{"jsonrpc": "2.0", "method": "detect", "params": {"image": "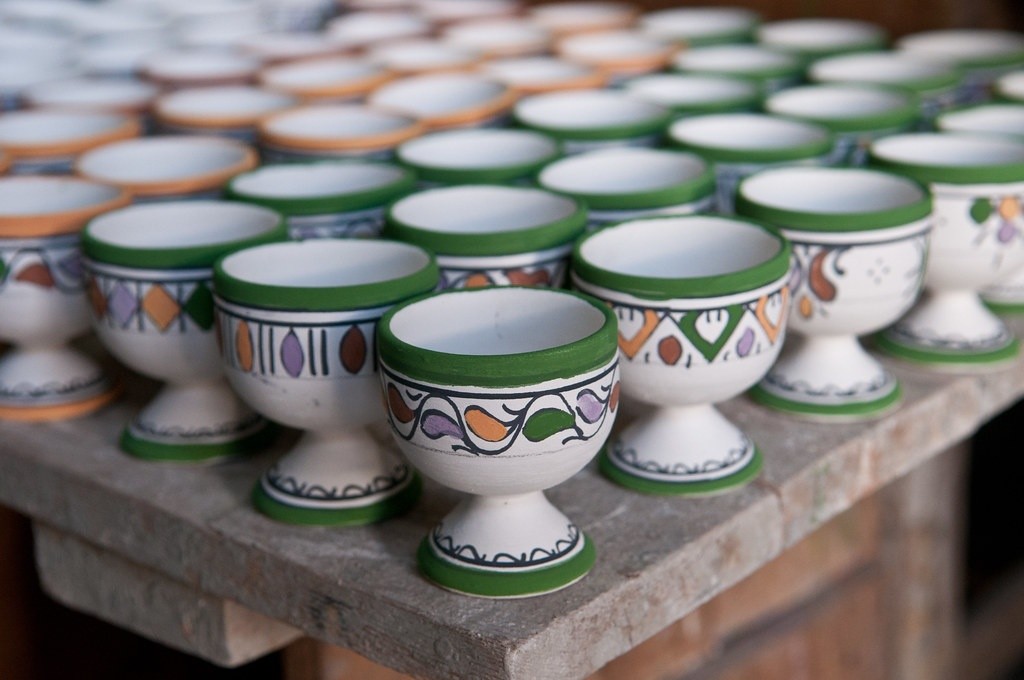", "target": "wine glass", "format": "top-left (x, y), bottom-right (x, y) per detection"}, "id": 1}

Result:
top-left (0, 0), bottom-right (1024, 599)
